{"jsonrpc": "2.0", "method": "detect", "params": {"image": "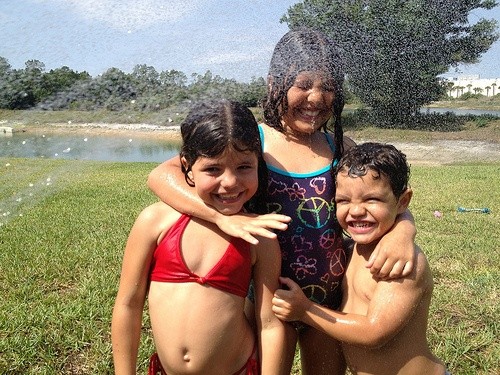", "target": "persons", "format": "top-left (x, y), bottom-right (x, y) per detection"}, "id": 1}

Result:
top-left (146, 27), bottom-right (417, 375)
top-left (271, 142), bottom-right (446, 375)
top-left (111, 99), bottom-right (285, 375)
